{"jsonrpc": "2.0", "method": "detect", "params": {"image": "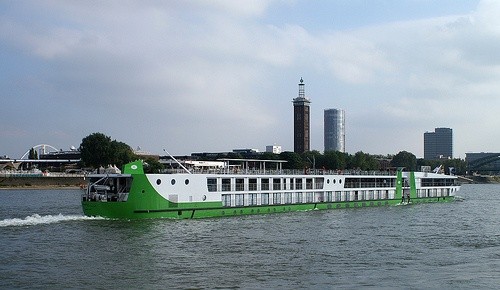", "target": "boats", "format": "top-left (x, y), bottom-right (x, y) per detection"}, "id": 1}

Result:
top-left (80, 162), bottom-right (461, 220)
